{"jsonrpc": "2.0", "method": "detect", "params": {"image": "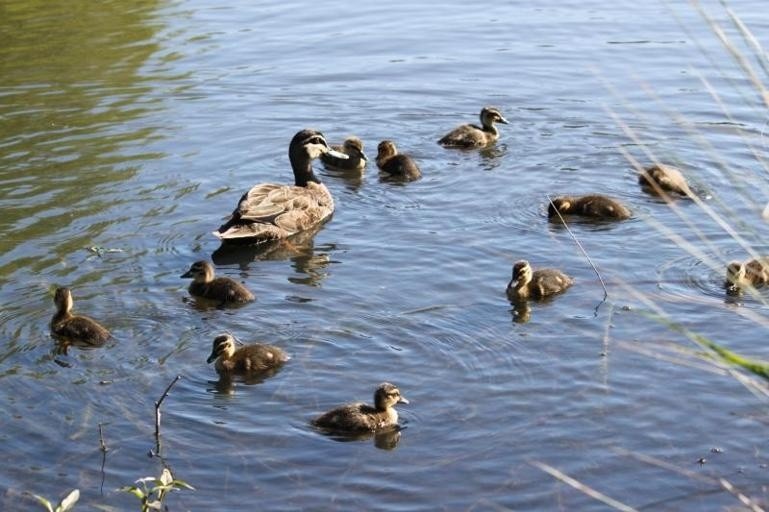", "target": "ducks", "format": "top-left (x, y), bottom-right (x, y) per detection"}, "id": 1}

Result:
top-left (638, 164), bottom-right (704, 200)
top-left (206, 372), bottom-right (279, 396)
top-left (546, 194), bottom-right (632, 221)
top-left (725, 256), bottom-right (768, 292)
top-left (505, 298), bottom-right (559, 324)
top-left (319, 135), bottom-right (422, 180)
top-left (311, 382), bottom-right (410, 433)
top-left (329, 423), bottom-right (406, 450)
top-left (49, 285), bottom-right (114, 350)
top-left (180, 259), bottom-right (257, 305)
top-left (204, 332), bottom-right (293, 373)
top-left (505, 260), bottom-right (574, 298)
top-left (435, 105), bottom-right (511, 150)
top-left (210, 127), bottom-right (351, 247)
